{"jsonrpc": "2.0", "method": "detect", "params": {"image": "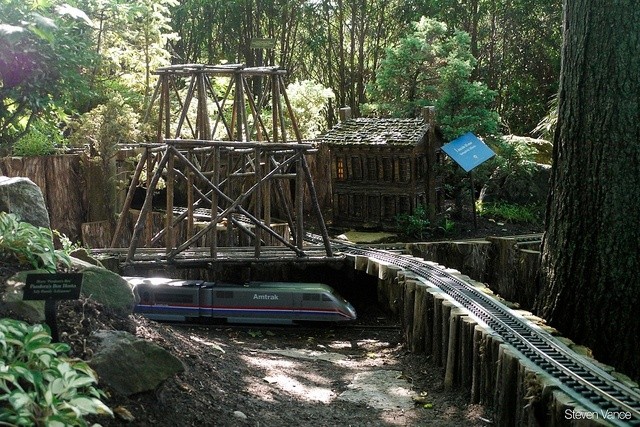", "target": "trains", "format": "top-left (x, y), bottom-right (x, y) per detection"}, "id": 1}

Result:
top-left (118, 276), bottom-right (357, 325)
